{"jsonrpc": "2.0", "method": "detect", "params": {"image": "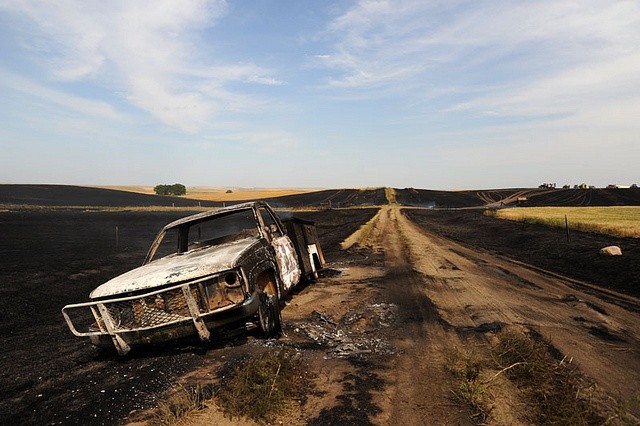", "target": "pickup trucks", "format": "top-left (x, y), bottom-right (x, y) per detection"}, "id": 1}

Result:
top-left (63, 202), bottom-right (328, 355)
